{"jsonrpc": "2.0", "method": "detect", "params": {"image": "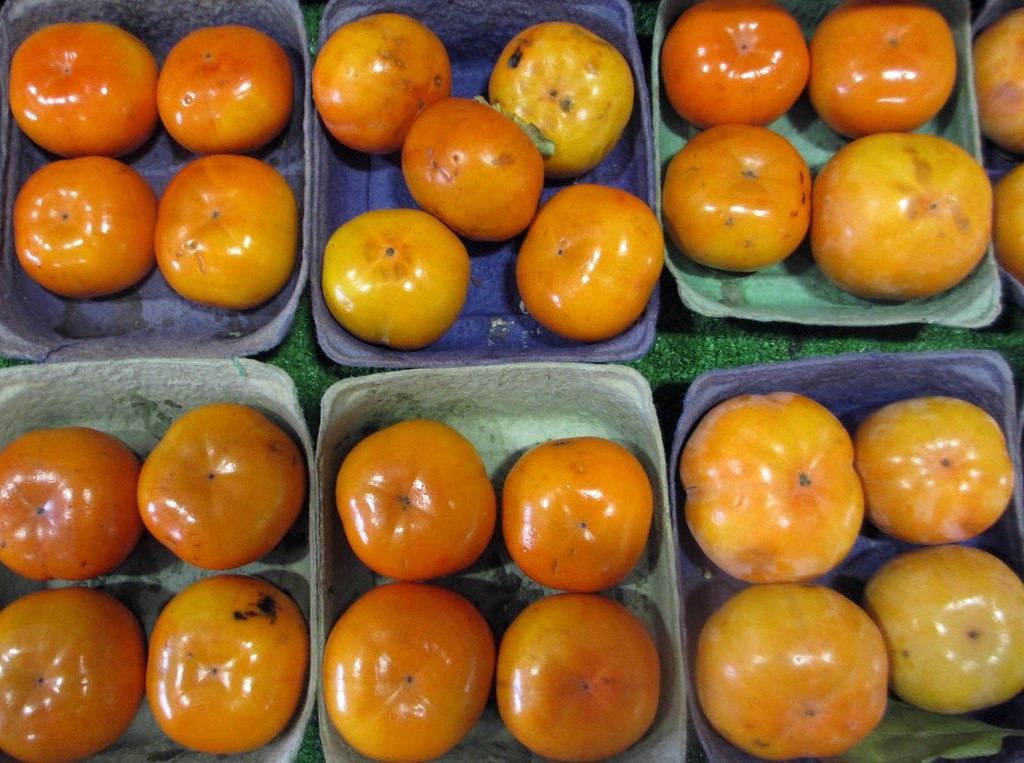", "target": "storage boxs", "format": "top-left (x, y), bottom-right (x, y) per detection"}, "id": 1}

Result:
top-left (0, 0), bottom-right (1024, 763)
